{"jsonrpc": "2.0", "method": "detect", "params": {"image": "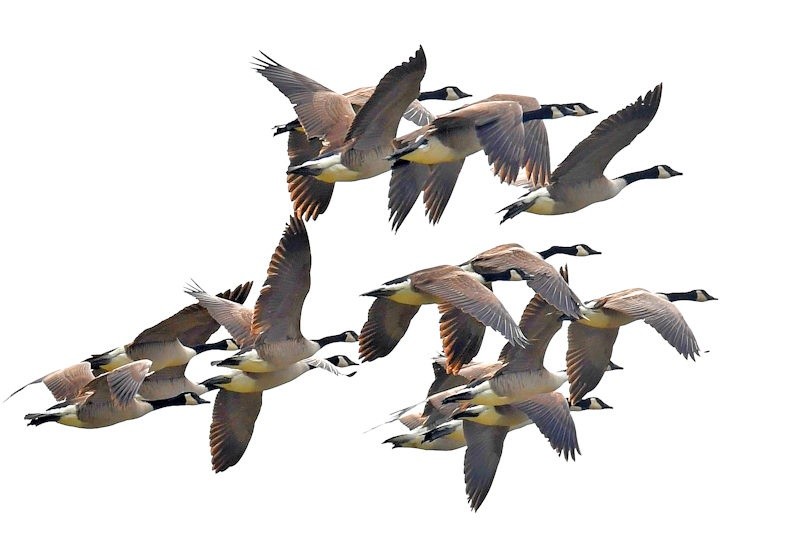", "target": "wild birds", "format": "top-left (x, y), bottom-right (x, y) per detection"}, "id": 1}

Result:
top-left (0, 210), bottom-right (359, 474)
top-left (248, 44), bottom-right (683, 234)
top-left (358, 243), bottom-right (719, 513)
